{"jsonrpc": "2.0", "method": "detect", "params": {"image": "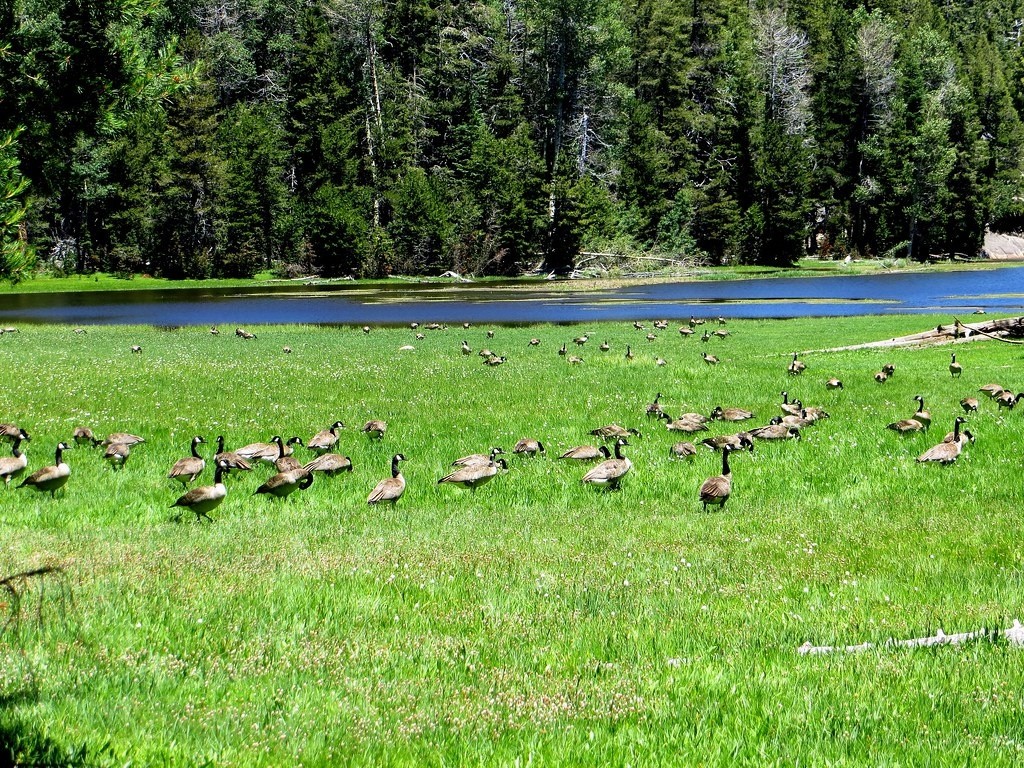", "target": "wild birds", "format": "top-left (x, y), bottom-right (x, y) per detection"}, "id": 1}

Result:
top-left (0, 314), bottom-right (1022, 525)
